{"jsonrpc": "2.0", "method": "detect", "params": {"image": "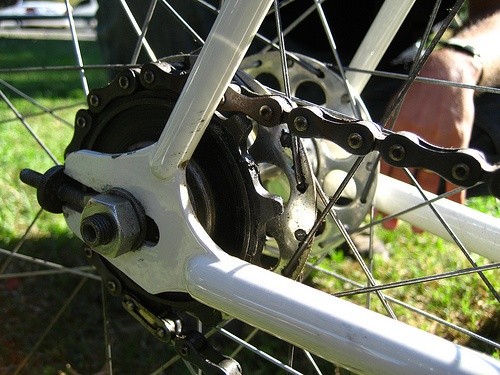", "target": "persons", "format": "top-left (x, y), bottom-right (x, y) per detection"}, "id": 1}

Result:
top-left (97, 1), bottom-right (500, 265)
